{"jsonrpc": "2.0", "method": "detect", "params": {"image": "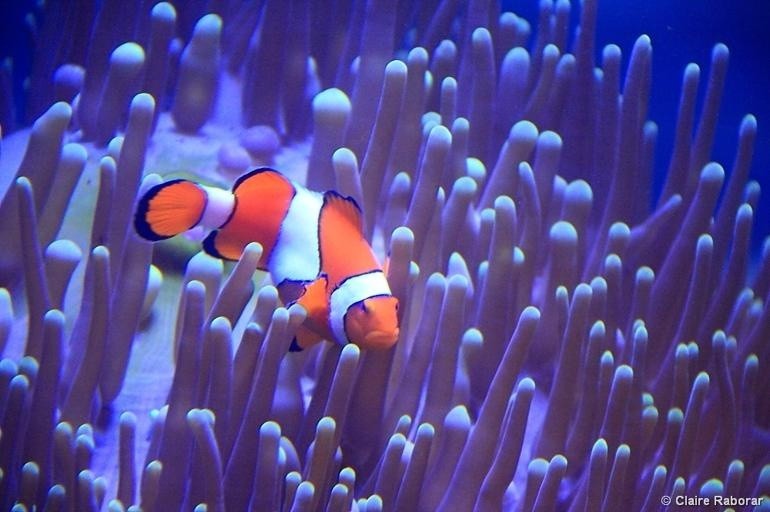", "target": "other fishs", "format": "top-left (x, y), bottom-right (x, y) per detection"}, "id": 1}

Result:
top-left (129, 163), bottom-right (405, 358)
